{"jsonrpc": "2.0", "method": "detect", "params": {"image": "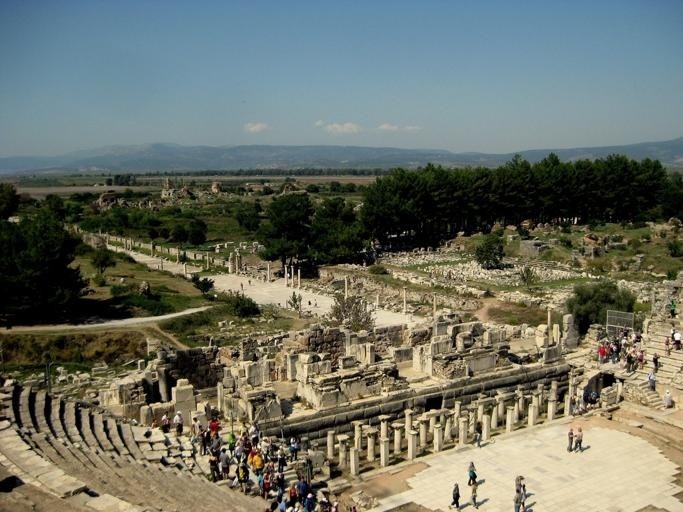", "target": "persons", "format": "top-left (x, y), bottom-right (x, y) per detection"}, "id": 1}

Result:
top-left (465, 460), bottom-right (476, 486)
top-left (515, 475), bottom-right (524, 493)
top-left (570, 300), bottom-right (683, 417)
top-left (445, 481), bottom-right (461, 511)
top-left (566, 428), bottom-right (575, 453)
top-left (573, 426), bottom-right (583, 452)
top-left (474, 421), bottom-right (482, 448)
top-left (471, 482), bottom-right (479, 509)
top-left (519, 483), bottom-right (527, 512)
top-left (149, 411), bottom-right (358, 512)
top-left (512, 489), bottom-right (522, 512)
